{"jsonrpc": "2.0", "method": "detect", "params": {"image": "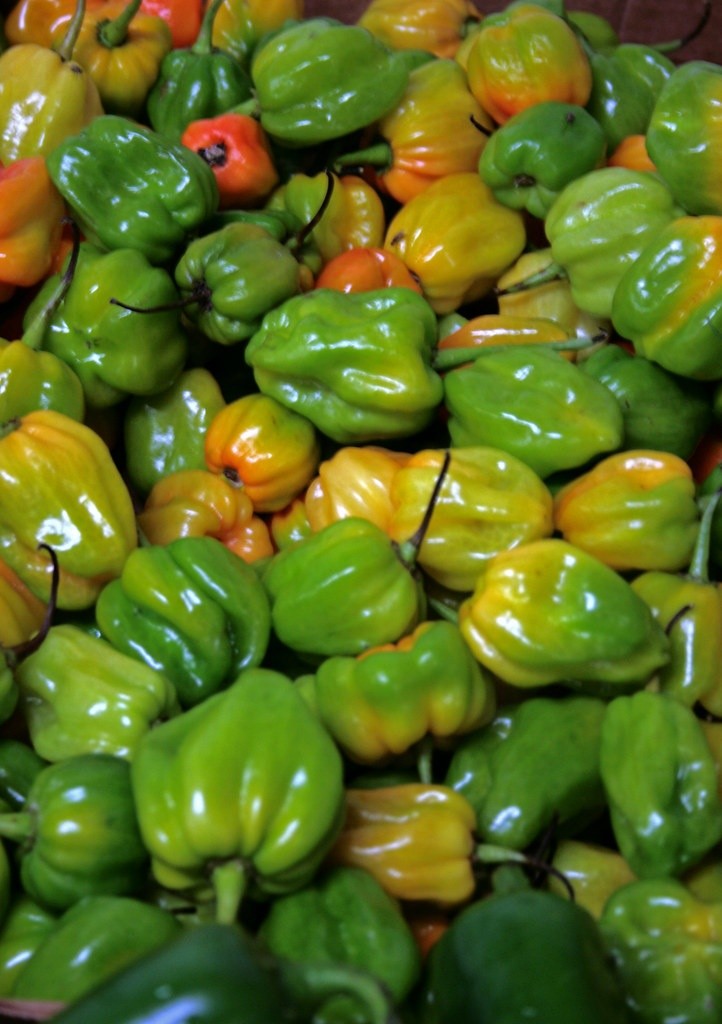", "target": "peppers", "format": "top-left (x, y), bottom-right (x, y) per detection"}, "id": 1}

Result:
top-left (0, 0), bottom-right (722, 1024)
